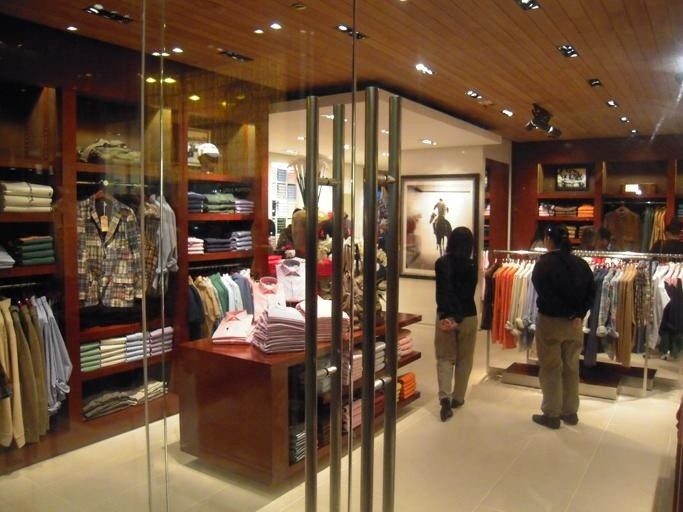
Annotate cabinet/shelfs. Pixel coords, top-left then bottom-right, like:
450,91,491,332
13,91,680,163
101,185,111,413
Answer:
533,160,683,254
177,305,423,485
1,80,272,479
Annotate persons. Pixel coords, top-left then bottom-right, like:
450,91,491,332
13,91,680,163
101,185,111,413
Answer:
278,208,303,250
434,198,449,220
435,227,478,422
532,225,595,428
527,226,618,253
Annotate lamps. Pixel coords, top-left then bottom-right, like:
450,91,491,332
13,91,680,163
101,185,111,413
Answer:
525,104,563,138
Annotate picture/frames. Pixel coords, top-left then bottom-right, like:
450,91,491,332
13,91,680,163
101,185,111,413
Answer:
397,172,480,276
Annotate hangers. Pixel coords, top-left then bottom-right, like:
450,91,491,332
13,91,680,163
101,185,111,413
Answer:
486,250,683,285
95,178,149,203
7,282,43,307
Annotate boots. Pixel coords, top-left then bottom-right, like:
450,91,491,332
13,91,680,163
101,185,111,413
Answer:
441,398,464,421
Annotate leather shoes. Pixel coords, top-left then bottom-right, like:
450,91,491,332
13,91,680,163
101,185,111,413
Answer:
533,414,578,428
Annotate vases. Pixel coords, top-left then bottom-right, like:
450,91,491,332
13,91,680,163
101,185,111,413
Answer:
290,207,329,258
